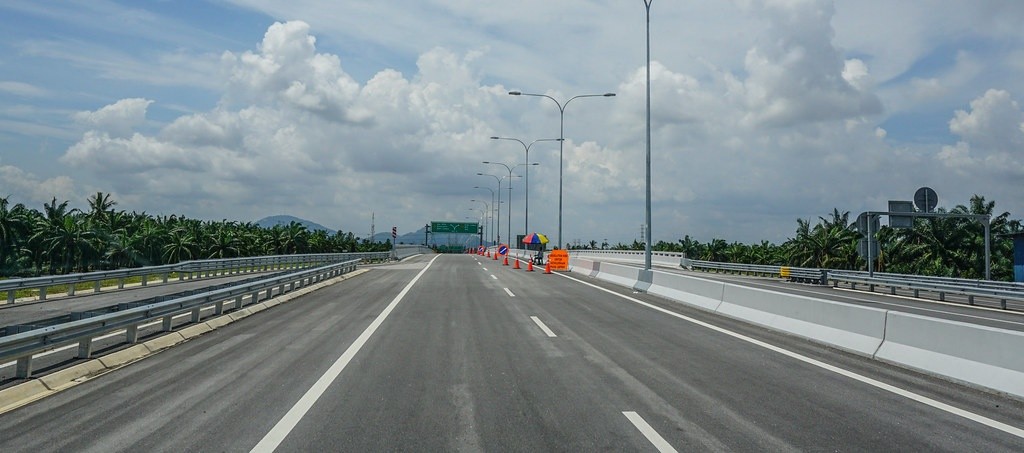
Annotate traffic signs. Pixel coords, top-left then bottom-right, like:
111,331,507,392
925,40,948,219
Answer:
431,221,479,234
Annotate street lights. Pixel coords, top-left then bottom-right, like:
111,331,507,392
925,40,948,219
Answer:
474,186,514,245
490,136,565,249
477,173,523,246
509,90,616,249
471,200,505,246
483,162,539,249
469,208,498,245
465,216,492,245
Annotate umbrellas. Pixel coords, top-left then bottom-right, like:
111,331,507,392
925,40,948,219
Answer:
522,232,549,265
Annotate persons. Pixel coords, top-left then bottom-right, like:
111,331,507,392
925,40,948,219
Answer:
532,247,543,265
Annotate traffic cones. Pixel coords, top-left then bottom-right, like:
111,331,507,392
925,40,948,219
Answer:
486,247,491,257
492,248,499,260
542,254,555,274
502,251,510,266
468,247,485,256
512,253,522,269
526,254,536,272
